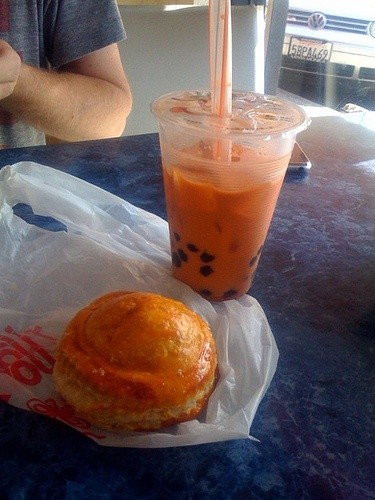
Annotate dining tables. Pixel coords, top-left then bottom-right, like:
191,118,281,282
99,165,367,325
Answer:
0,111,375,500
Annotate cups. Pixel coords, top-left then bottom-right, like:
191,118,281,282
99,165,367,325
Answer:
151,88,311,302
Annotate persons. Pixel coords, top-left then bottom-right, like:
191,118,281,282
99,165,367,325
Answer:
0,0,131,149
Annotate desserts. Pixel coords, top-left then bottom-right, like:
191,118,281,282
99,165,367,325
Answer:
54,289,219,431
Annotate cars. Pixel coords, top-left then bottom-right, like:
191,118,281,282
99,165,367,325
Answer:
272,2,375,97
